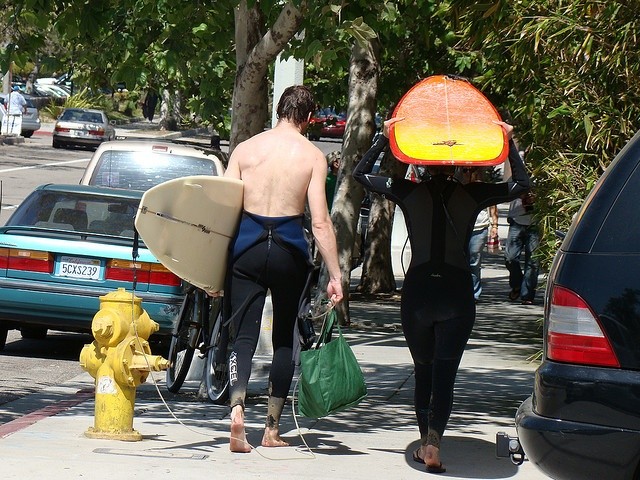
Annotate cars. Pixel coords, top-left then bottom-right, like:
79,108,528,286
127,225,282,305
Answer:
0,183,200,349
52,107,116,147
306,107,347,141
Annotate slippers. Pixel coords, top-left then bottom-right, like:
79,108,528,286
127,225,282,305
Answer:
522,297,533,304
509,284,521,301
412,447,424,461
426,461,446,473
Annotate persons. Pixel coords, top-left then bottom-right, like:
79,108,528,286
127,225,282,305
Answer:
144,89,162,124
351,117,530,474
326,151,342,213
3,85,27,136
206,86,345,453
359,134,386,260
503,196,541,305
468,203,499,304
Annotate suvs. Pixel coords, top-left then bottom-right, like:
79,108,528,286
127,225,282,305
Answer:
496,130,640,479
76,139,228,224
0,94,41,137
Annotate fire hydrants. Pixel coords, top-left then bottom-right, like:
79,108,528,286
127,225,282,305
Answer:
80,287,172,441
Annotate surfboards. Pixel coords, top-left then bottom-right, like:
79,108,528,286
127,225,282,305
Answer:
389,73,510,166
134,175,244,293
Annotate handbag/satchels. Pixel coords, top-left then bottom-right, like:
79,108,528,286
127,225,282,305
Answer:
295,304,368,419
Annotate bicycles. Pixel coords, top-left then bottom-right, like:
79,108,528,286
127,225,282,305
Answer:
167,283,230,405
